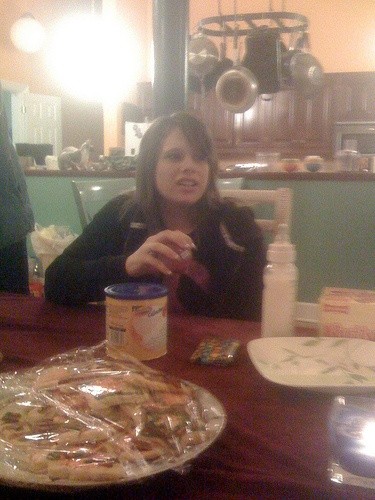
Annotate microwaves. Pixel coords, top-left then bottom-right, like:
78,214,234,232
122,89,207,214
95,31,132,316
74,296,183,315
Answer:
334,121,375,156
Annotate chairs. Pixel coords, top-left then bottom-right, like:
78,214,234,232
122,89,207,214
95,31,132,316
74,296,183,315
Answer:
73,176,292,238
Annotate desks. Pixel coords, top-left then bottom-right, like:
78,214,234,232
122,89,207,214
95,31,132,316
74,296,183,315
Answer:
0,291,375,500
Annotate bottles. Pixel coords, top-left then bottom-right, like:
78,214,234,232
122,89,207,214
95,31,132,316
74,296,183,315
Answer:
259,223,298,337
338,149,361,172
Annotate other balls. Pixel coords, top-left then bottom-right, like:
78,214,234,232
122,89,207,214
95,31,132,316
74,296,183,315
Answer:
302,155,324,172
157,240,193,273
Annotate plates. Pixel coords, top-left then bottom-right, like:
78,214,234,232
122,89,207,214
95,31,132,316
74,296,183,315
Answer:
0,378,226,488
246,336,375,395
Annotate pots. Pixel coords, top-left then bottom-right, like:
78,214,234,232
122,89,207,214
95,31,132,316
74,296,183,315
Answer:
188,23,324,115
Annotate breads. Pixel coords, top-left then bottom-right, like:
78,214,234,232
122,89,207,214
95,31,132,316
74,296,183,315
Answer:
0,357,211,481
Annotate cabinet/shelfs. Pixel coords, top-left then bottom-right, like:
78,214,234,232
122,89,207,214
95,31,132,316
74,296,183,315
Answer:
137,71,375,162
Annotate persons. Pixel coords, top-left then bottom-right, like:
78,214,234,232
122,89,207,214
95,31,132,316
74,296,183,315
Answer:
44,112,266,319
0,98,35,296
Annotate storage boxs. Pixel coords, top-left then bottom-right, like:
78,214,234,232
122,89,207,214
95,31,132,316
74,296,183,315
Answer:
318,287,375,341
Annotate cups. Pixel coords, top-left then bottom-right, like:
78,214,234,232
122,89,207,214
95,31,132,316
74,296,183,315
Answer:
327,395,375,489
255,152,322,172
